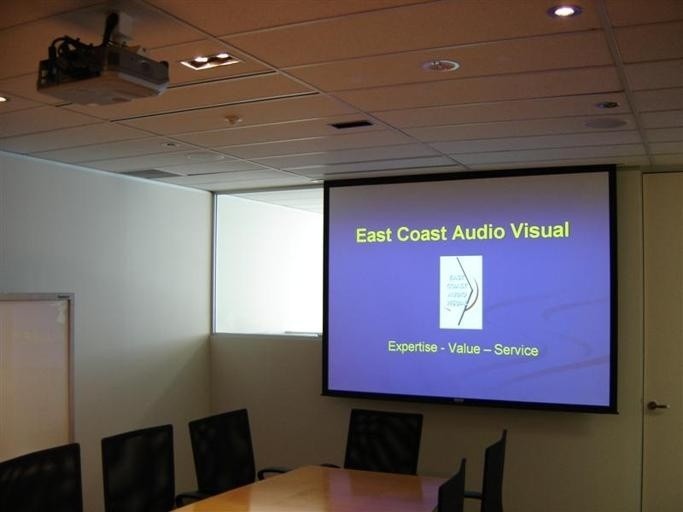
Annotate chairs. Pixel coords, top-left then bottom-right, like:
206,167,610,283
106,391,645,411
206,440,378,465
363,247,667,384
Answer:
101,424,200,512
437,458,466,512
321,408,424,476
463,427,508,512
187,408,293,495
0,442,83,512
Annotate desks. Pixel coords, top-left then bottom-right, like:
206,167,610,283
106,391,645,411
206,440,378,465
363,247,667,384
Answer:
173,465,451,512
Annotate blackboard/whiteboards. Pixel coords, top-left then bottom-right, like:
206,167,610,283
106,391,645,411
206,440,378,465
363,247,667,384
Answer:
0,292,76,465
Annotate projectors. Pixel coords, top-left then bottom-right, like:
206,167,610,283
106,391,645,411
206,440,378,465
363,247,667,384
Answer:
35,12,170,106
